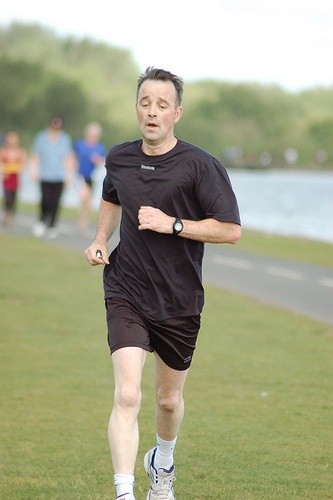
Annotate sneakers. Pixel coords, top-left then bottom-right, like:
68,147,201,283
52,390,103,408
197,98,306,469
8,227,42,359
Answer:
143,447,176,500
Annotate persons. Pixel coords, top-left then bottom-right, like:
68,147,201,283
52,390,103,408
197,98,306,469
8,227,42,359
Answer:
30,115,76,240
0,130,27,225
86,67,241,500
73,123,106,235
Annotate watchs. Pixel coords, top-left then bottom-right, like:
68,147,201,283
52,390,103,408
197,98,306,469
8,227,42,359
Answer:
172,217,184,235
